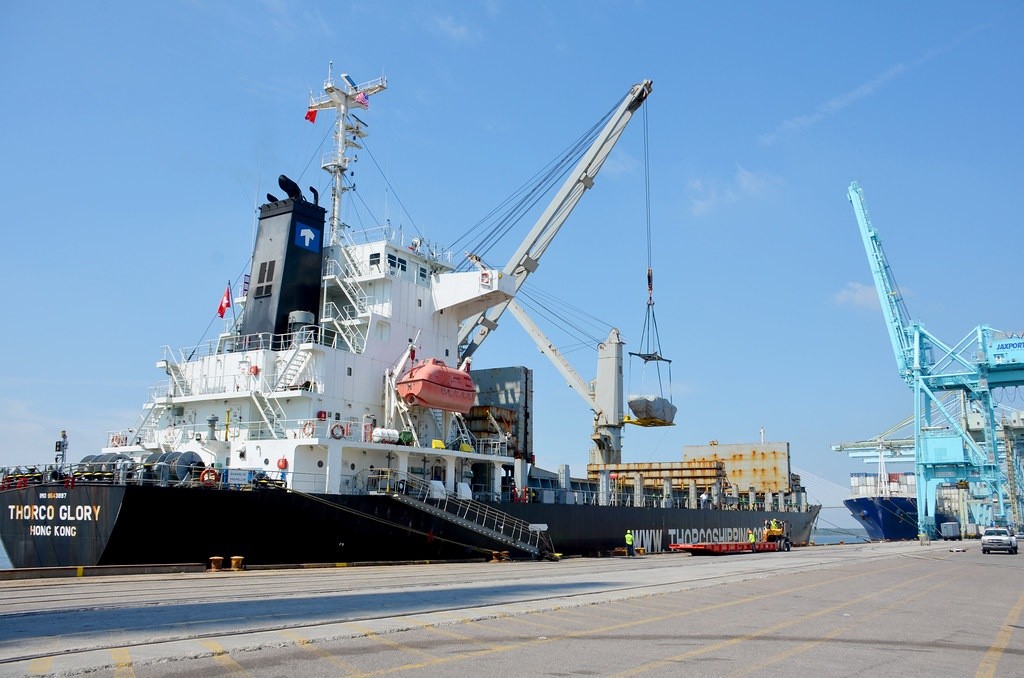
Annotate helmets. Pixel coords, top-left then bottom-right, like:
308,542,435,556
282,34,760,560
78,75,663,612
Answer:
627,530,632,533
748,530,752,533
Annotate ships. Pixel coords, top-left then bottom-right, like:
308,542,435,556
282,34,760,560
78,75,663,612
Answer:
0,60,823,569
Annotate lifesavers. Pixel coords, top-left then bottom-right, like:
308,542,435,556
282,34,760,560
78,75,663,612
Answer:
200,467,219,487
301,421,316,437
330,424,345,440
110,434,121,447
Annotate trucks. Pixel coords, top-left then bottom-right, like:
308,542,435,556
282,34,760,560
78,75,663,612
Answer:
668,521,792,555
967,524,986,539
941,522,962,541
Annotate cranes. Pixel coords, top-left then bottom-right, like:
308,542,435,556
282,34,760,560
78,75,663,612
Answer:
830,180,1024,538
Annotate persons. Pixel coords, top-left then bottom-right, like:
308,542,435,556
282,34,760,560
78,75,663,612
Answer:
700,491,708,509
625,530,635,557
747,530,757,553
772,518,777,529
714,493,718,510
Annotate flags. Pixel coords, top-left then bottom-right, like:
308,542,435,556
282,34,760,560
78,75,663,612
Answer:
305,110,318,123
218,288,231,318
357,92,369,106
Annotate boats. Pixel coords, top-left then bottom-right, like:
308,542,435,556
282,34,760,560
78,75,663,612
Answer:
396,357,477,413
842,496,982,541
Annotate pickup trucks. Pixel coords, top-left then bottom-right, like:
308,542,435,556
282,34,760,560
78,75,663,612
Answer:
981,525,1018,554
